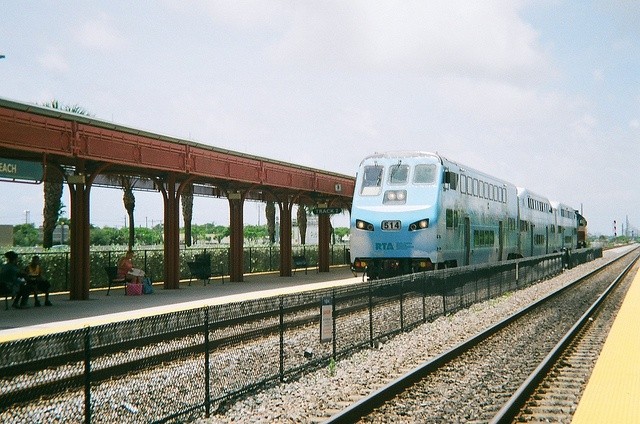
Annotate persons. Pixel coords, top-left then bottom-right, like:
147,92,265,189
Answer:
24,255,53,307
0,251,29,309
117,250,143,284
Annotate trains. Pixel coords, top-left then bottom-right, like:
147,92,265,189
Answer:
350,151,587,281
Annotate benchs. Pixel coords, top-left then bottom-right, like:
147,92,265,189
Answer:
189,262,225,287
105,265,153,296
1,274,49,309
292,256,319,276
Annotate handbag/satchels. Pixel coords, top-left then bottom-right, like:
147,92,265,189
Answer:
143,278,153,294
